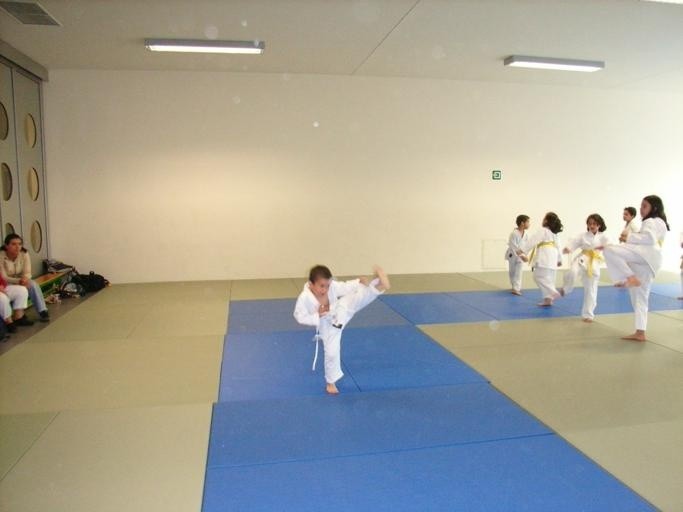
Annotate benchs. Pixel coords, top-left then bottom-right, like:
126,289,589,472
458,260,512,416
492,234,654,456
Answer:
29,267,79,297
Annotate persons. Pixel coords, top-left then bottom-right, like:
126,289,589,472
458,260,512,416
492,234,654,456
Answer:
677,241,683,299
603,196,670,341
293,265,391,394
619,207,639,244
0,274,34,333
560,214,606,323
517,212,564,306
0,234,49,321
505,215,529,295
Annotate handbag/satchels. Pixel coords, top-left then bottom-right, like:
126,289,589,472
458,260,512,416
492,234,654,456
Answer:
72,272,108,293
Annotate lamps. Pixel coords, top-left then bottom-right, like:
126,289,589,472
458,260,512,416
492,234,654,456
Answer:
142,37,265,58
503,56,605,74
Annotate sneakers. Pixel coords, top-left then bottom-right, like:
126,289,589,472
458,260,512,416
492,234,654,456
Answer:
6,311,51,333
